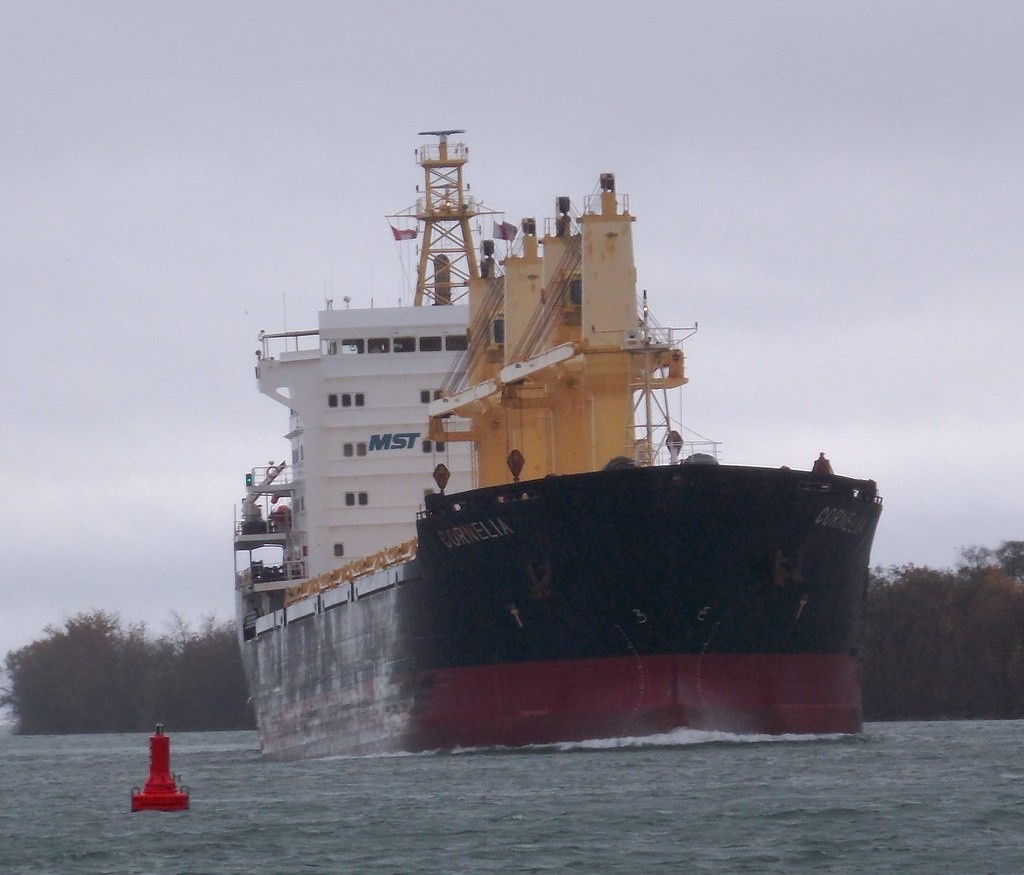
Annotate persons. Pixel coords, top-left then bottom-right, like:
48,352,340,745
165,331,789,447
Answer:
812,453,834,475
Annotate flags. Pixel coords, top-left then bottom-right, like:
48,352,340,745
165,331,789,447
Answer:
493,222,517,241
391,225,416,240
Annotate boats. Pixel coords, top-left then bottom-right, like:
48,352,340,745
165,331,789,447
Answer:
229,126,887,765
